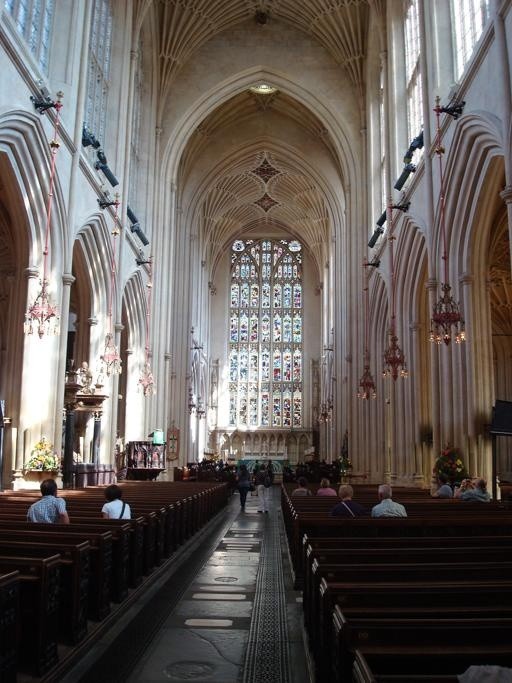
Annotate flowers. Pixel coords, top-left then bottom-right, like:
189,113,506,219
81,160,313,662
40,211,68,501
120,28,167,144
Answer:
18,435,62,473
432,448,467,482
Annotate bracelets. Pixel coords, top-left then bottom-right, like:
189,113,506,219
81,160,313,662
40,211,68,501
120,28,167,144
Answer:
459,484,464,487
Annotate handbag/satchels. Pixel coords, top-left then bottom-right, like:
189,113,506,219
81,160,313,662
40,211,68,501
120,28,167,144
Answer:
248,484,256,492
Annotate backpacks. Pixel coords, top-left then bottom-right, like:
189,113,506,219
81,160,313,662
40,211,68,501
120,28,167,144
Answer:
263,475,272,488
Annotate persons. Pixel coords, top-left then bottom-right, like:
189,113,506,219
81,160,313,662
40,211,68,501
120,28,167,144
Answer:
454,478,490,502
428,474,453,498
370,484,408,517
317,478,338,496
332,485,364,518
102,484,132,520
26,479,71,525
292,478,311,495
190,456,276,515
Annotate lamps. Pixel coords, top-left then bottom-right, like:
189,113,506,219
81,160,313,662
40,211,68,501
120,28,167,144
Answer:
90,190,127,376
80,127,150,247
381,197,415,382
131,249,157,397
430,90,476,344
356,259,382,402
187,345,206,418
316,346,335,424
367,130,424,250
23,86,68,341
